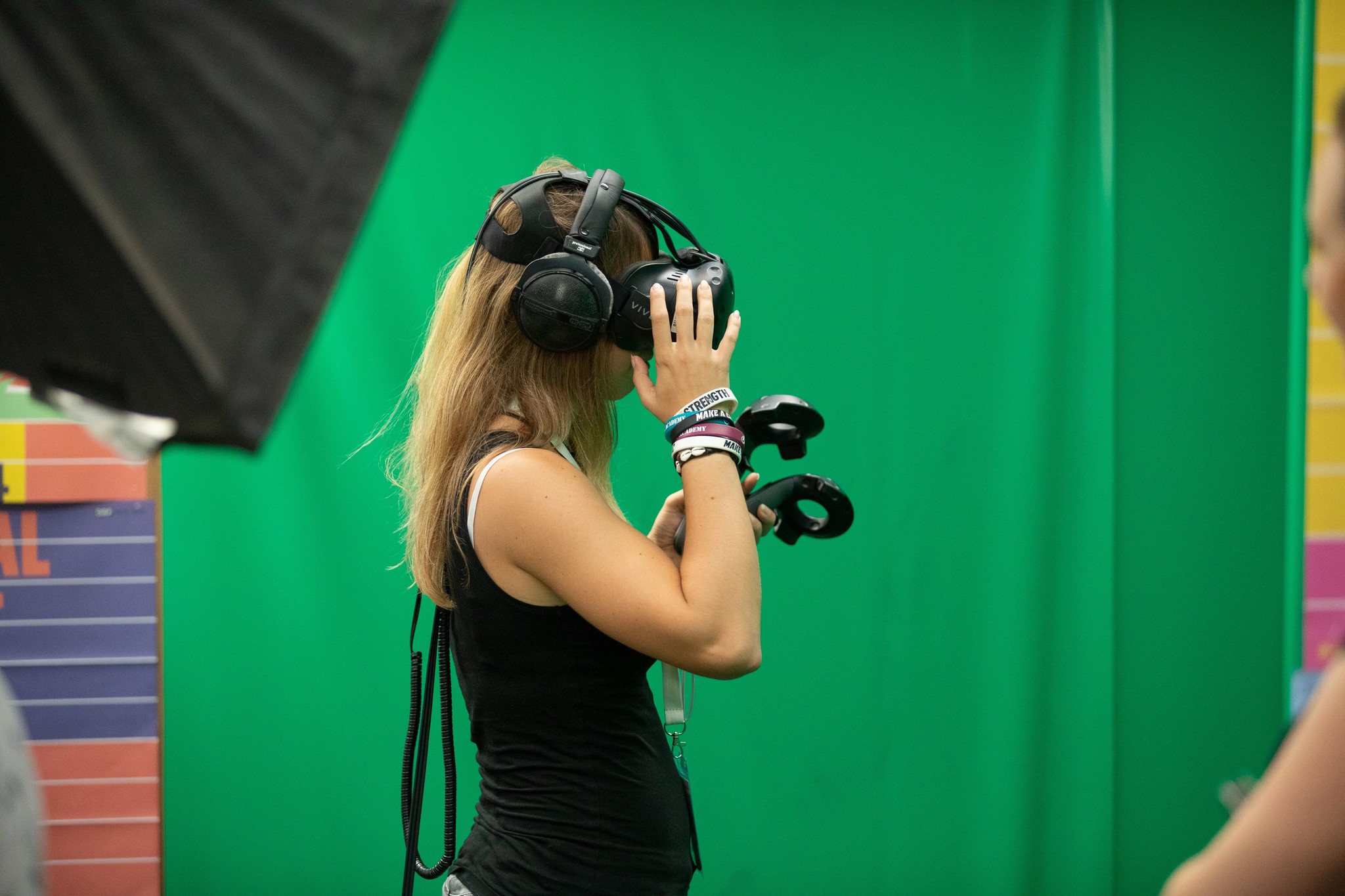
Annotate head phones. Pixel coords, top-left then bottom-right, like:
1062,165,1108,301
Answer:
510,167,625,353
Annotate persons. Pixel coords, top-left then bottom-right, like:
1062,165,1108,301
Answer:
1149,95,1345,895
404,163,776,896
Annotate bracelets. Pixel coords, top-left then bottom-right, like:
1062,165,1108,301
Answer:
663,388,745,474
